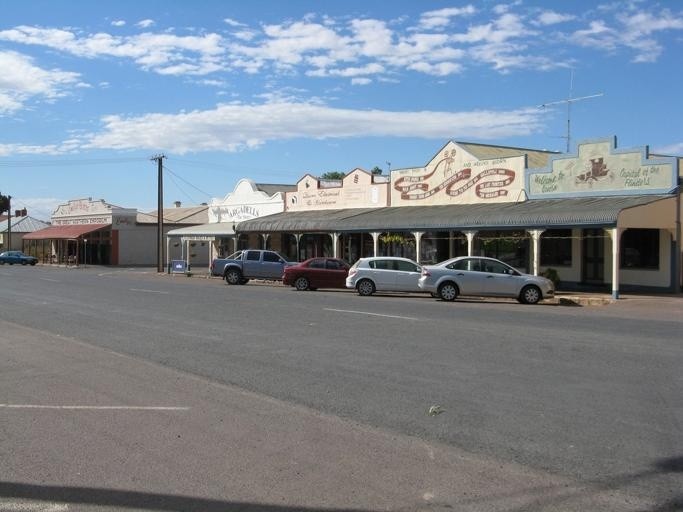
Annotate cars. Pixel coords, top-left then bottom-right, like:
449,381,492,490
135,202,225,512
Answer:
0,251,38,265
